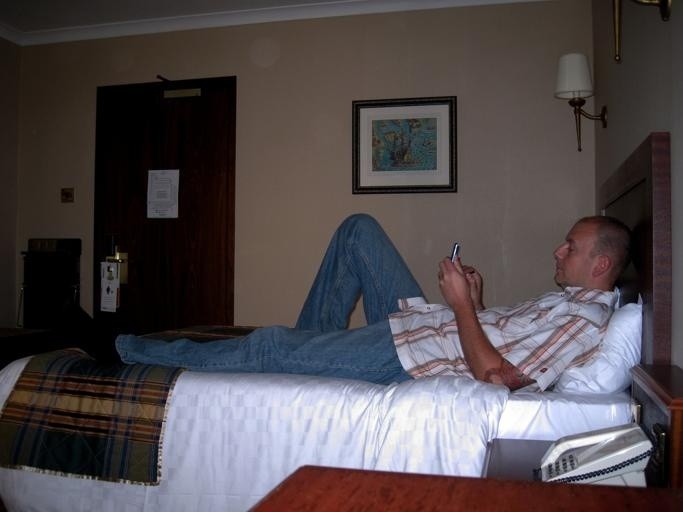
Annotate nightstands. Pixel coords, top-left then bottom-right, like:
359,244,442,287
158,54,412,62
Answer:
486,438,658,494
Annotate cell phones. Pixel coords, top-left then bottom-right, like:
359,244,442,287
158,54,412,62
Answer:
451,243,460,262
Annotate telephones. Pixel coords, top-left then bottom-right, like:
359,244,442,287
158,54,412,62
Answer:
540,422,654,487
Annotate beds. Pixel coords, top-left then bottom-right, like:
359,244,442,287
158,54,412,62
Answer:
0,133,670,512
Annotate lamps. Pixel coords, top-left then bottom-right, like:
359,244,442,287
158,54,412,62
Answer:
555,52,607,153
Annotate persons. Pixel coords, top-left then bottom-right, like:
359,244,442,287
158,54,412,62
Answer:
54,211,634,394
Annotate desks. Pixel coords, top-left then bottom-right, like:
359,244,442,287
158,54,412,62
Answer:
251,467,676,511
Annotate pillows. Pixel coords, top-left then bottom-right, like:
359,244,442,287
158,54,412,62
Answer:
552,293,644,397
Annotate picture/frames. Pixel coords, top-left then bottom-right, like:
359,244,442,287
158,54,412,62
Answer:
350,96,459,194
